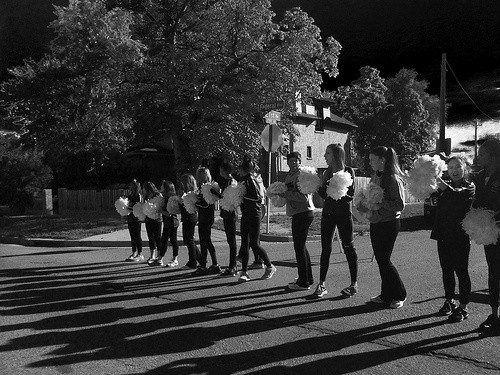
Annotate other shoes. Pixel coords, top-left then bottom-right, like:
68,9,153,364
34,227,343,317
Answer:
448,306,469,322
479,313,500,335
248,262,264,268
261,264,276,280
438,299,457,315
126,253,145,261
288,282,310,290
370,295,389,303
312,283,327,298
341,285,359,298
152,259,163,265
192,265,221,276
166,259,178,267
389,297,406,308
181,264,197,269
237,271,250,282
216,268,239,277
293,278,315,284
147,256,158,263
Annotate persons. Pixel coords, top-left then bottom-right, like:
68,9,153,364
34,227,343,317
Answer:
429,156,475,323
116,155,276,283
274,152,313,289
357,146,408,308
314,144,359,298
471,138,500,334
